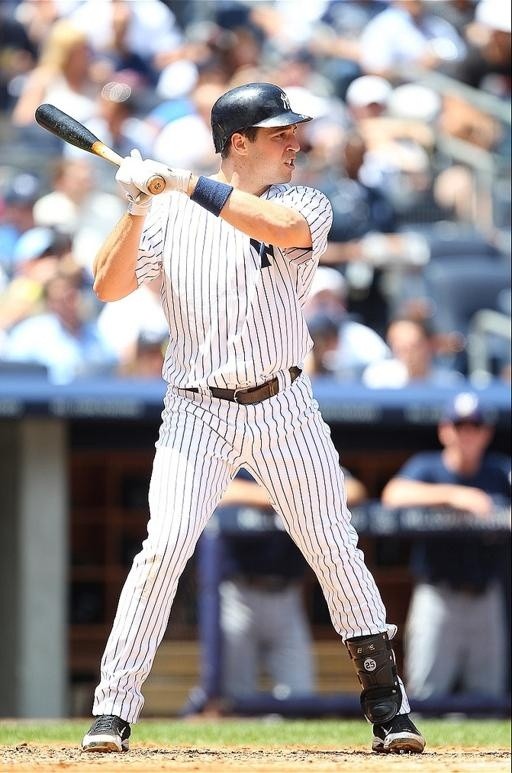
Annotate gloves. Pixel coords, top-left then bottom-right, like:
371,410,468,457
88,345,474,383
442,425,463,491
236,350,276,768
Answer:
132,158,193,196
114,148,154,216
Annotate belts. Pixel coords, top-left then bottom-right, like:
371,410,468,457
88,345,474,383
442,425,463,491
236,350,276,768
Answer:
178,366,303,405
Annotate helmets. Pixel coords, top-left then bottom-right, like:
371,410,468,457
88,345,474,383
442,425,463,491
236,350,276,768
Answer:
209,82,315,153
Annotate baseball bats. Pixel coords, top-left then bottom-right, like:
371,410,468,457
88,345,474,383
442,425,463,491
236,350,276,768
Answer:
35,104,166,194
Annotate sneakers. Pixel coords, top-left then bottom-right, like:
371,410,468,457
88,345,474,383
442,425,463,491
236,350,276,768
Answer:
81,714,131,754
371,713,426,754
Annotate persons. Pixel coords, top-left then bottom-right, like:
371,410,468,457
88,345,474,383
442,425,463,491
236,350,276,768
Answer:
3,0,510,388
207,461,366,701
377,387,511,718
84,79,429,753
